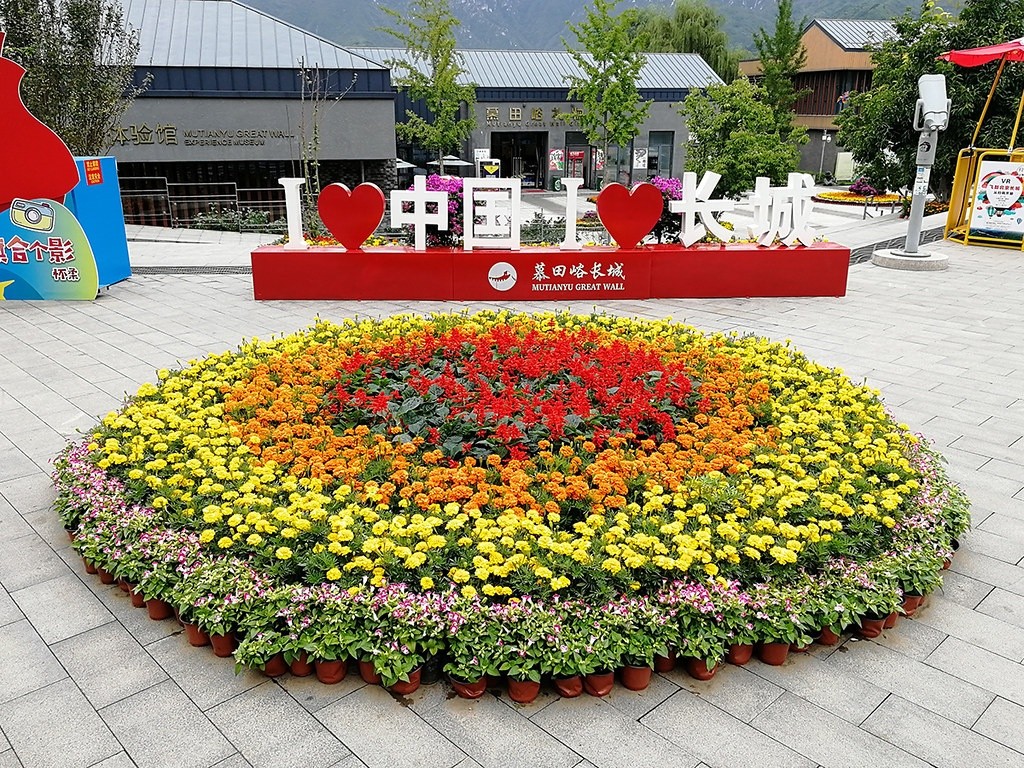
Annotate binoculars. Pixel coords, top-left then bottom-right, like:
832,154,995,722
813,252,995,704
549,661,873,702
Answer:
918,74,948,132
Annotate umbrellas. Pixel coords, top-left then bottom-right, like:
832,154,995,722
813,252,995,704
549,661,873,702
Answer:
427,155,474,165
396,157,417,168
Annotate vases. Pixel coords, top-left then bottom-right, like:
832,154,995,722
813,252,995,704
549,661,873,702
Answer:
72,532,949,702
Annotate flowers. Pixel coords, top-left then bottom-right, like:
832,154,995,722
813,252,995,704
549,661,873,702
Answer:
172,174,799,246
50,310,975,685
809,179,950,220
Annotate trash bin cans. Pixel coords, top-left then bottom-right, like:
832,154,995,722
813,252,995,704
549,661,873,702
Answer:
552,176,560,192
597,176,603,191
